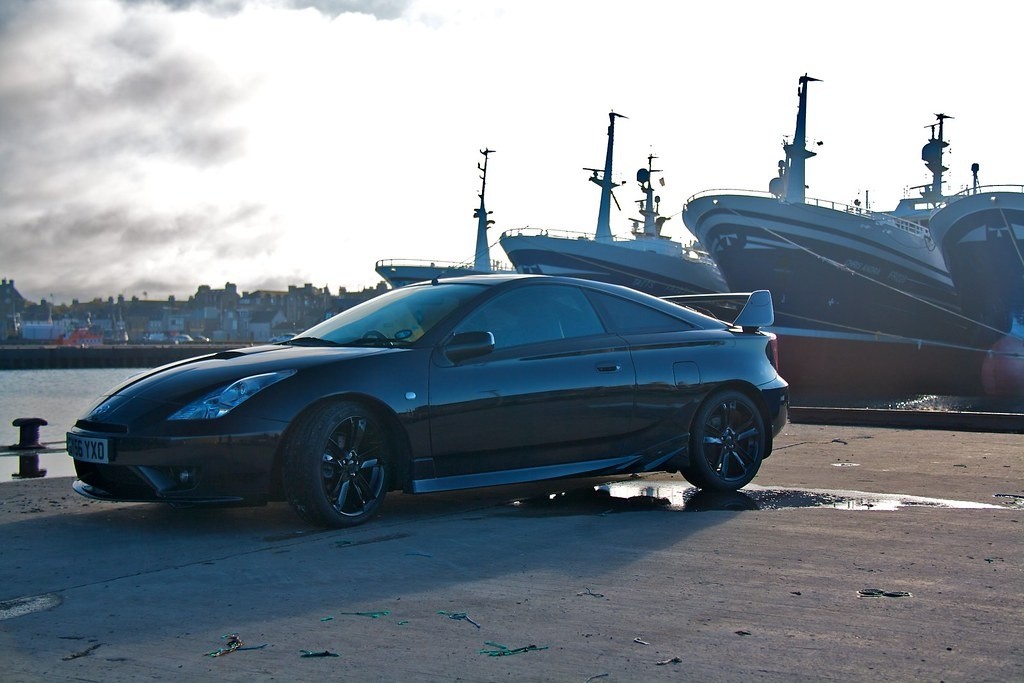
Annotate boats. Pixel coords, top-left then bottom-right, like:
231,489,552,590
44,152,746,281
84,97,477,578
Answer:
374,73,1023,409
46,322,106,344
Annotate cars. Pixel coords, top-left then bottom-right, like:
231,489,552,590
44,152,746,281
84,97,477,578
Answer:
268,334,296,343
192,335,211,342
170,335,195,343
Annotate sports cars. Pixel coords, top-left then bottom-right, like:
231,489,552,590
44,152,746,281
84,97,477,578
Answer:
65,271,789,528
